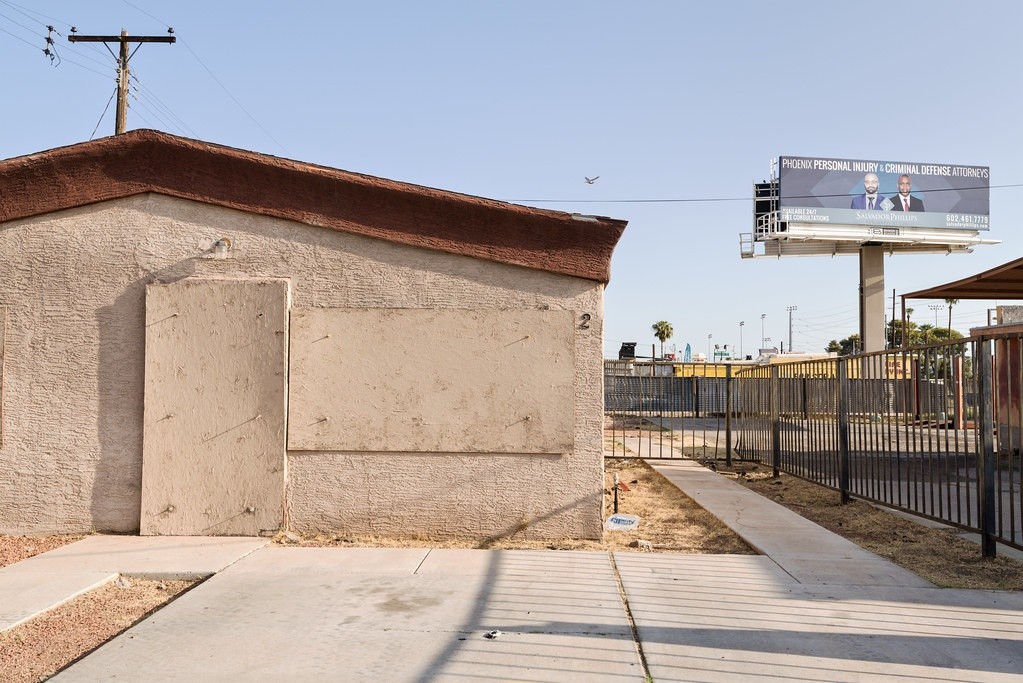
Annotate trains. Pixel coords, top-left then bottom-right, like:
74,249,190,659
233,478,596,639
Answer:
603,375,949,418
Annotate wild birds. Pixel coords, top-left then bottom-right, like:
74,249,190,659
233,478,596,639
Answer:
584,176,599,184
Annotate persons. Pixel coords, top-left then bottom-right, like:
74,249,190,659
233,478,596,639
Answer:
850,172,890,212
887,173,925,212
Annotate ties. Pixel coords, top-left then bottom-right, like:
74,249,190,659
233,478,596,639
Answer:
904,199,910,211
868,197,875,210
